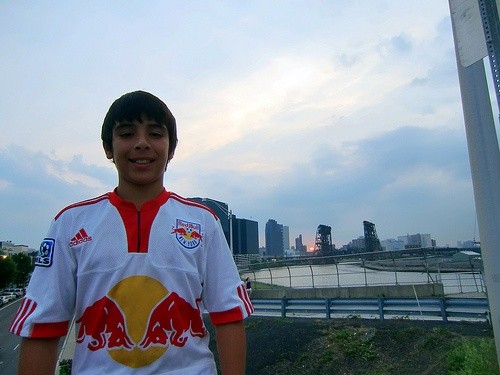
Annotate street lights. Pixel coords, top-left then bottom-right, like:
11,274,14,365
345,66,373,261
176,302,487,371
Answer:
201,197,234,257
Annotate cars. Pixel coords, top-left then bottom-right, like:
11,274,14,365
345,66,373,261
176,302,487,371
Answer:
2,292,16,300
0,295,8,303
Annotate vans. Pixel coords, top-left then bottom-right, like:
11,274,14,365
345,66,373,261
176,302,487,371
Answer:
4,288,23,297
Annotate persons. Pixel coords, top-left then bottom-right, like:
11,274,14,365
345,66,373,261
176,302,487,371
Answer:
7,91,255,375
245,277,253,301
